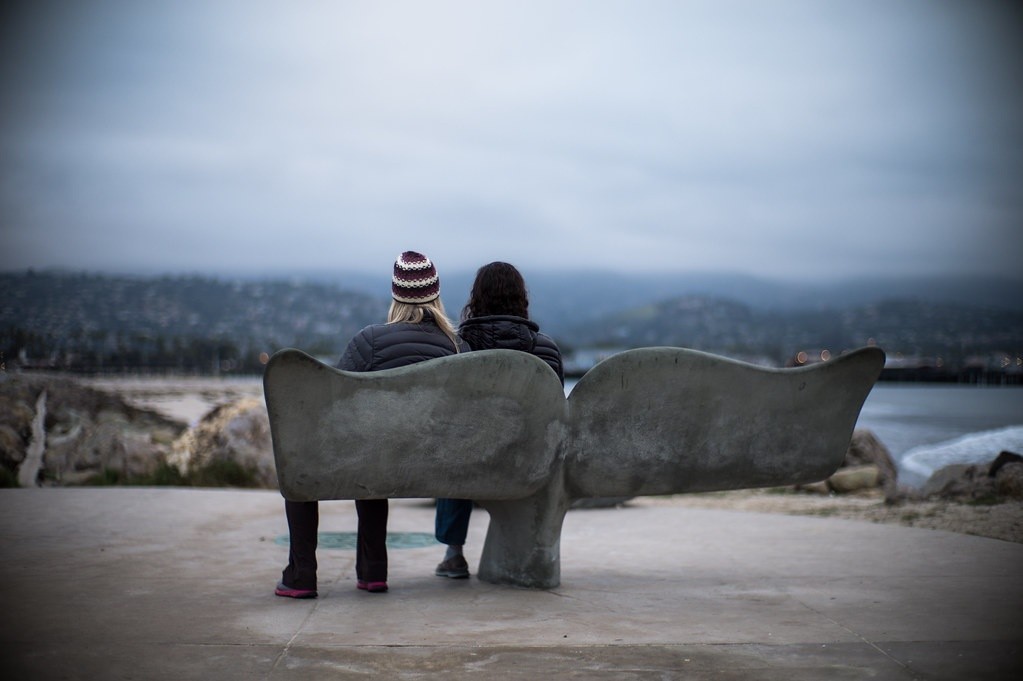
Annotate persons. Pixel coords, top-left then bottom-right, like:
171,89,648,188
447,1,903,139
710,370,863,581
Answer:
434,261,563,577
274,250,471,600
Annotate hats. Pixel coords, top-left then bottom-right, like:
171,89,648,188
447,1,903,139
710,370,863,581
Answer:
391,252,440,303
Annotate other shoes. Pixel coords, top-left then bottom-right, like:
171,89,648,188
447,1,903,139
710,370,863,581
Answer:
435,555,468,579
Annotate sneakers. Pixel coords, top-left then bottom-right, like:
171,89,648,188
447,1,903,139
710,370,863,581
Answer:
274,580,318,600
356,571,388,593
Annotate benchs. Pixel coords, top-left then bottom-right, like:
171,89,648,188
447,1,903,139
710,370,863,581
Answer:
262,348,885,591
